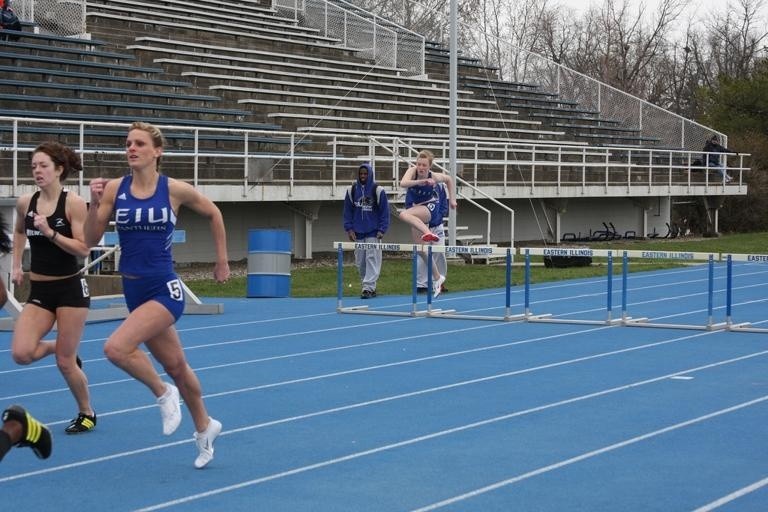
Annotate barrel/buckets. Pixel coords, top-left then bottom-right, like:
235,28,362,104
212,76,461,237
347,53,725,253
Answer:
246,226,293,297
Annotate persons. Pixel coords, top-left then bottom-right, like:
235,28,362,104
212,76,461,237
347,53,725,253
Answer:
398,149,458,299
342,163,390,299
83,121,231,468
0,0,14,41
0,208,52,462
703,135,738,183
11,140,96,432
405,182,450,293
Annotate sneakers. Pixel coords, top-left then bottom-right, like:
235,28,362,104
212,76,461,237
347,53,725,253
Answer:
432,274,446,298
193,416,222,468
156,381,182,436
1,403,53,459
65,408,97,434
360,291,377,299
421,233,439,242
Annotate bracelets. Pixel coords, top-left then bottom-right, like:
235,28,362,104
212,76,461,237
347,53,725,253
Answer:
52,230,59,242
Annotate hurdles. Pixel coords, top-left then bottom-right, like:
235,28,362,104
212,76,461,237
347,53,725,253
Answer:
85,234,225,321
333,240,768,335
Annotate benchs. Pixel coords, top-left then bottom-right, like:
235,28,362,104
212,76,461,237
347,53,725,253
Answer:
0,0,711,185
